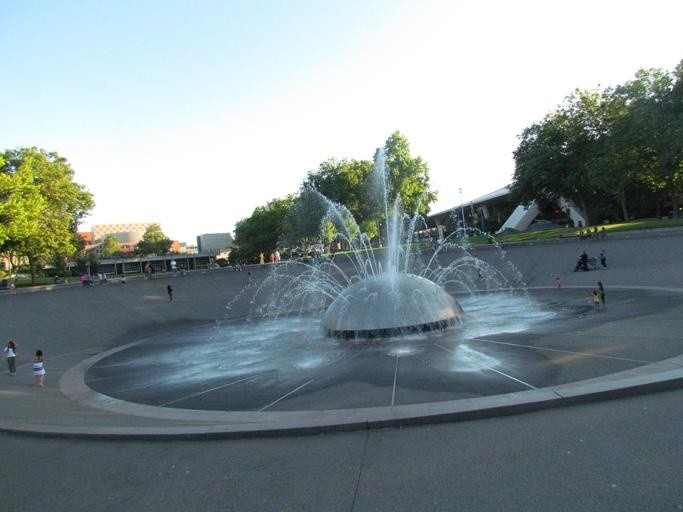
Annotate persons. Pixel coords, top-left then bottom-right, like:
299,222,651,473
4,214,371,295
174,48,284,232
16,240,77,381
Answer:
555,277,561,289
268,242,324,264
32,350,47,386
598,281,605,304
592,290,600,311
79,271,127,287
144,260,185,279
579,227,607,241
573,250,607,272
3,340,20,376
168,284,174,303
